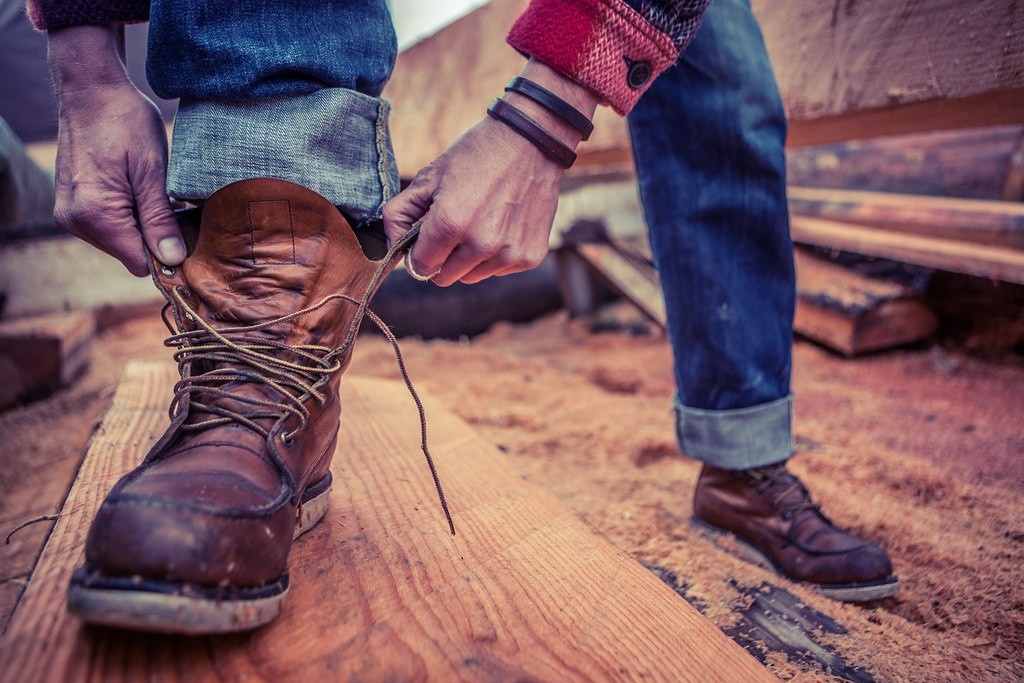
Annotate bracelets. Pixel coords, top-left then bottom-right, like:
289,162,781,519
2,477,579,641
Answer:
487,76,593,168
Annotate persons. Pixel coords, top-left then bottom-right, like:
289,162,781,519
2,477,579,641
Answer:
30,0,898,635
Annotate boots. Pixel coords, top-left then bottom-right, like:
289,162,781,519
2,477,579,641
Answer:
64,175,455,637
689,458,901,607
